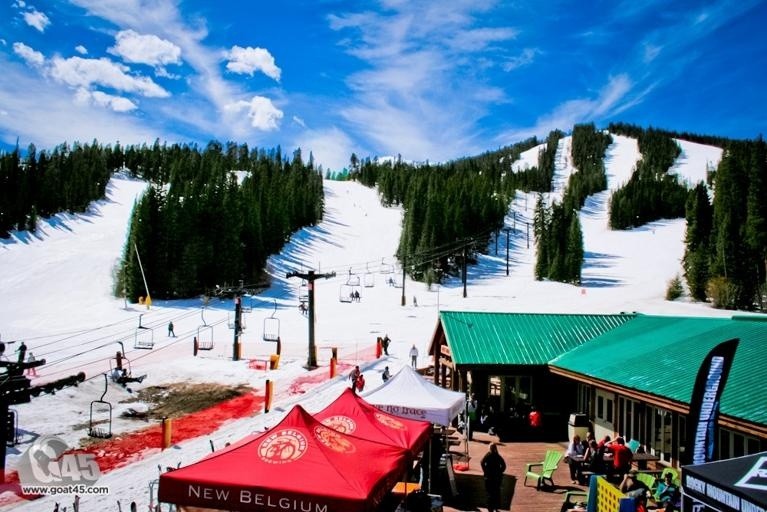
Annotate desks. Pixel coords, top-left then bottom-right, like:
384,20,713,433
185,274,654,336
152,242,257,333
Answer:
568,454,659,470
390,424,461,496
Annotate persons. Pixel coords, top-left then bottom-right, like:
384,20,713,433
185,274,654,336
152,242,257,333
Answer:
112,368,127,380
409,344,418,367
480,443,506,512
565,432,633,484
15,342,36,375
467,392,478,441
422,427,442,494
168,322,176,337
349,366,365,392
376,336,390,358
382,366,390,382
620,474,680,512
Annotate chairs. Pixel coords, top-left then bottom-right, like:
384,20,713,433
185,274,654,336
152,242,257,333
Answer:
524,448,564,491
565,468,681,512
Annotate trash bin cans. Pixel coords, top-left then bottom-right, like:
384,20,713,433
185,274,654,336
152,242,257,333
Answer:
568,413,590,443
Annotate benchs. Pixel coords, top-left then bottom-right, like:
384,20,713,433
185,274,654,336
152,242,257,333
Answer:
581,470,663,479
